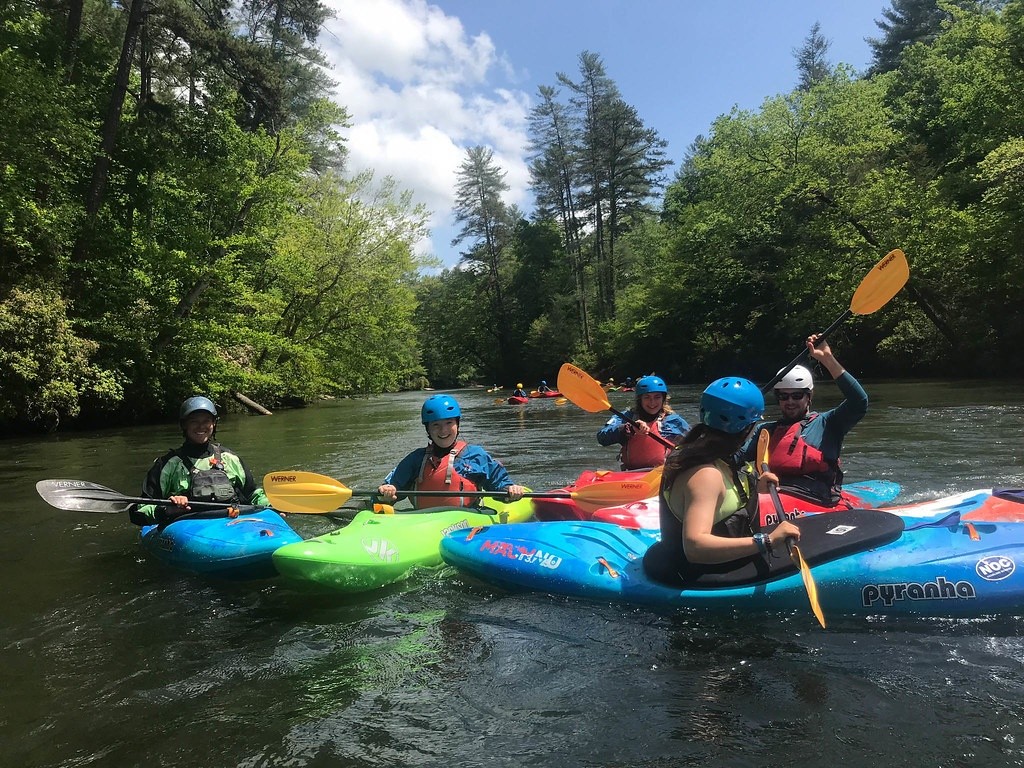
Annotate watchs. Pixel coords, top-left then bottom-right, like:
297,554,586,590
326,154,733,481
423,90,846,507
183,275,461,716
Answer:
753,533,766,553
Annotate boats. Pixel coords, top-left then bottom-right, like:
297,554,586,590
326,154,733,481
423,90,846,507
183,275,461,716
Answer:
589,471,901,543
506,396,528,406
493,388,500,392
139,498,307,577
440,489,1023,621
530,390,573,404
270,486,537,592
531,464,676,526
623,387,635,393
607,386,619,393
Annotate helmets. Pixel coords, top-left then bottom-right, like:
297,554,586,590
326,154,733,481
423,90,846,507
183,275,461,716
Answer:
634,376,667,399
517,383,523,389
179,396,217,425
699,376,765,433
773,365,813,394
541,381,546,387
642,375,647,378
421,394,462,423
609,378,615,382
627,377,631,382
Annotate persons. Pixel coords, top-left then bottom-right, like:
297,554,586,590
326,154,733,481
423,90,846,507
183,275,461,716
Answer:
493,384,497,389
129,396,287,526
659,377,801,565
734,334,869,486
597,376,692,472
624,377,632,388
605,377,614,388
377,394,523,510
512,382,527,397
537,381,552,393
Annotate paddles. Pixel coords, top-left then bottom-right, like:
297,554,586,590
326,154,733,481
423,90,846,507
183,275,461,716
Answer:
642,247,913,498
650,372,655,376
555,361,675,450
636,377,641,382
754,426,829,631
34,476,375,528
595,380,606,386
557,380,601,405
495,395,513,402
530,391,538,396
259,470,653,518
487,386,503,393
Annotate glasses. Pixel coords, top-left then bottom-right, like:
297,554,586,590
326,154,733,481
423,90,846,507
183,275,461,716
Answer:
778,392,807,402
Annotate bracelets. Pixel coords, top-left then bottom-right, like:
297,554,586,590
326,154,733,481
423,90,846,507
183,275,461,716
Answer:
764,534,780,567
